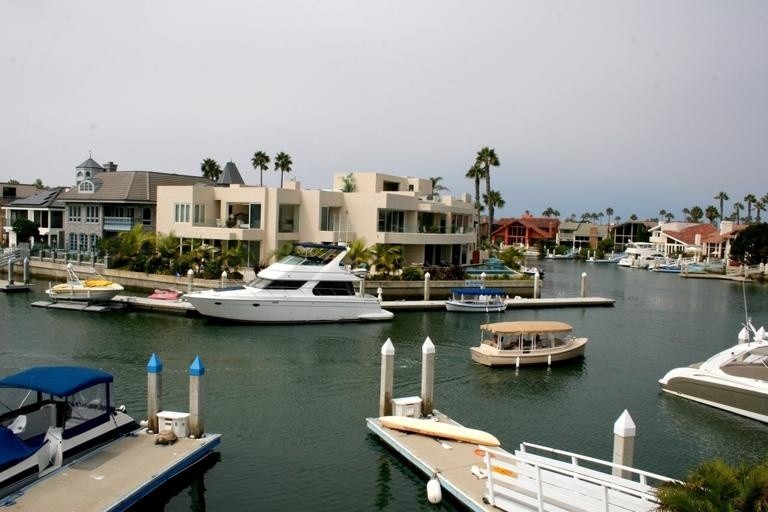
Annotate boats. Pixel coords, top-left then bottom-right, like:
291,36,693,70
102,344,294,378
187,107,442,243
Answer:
183,242,394,325
0,366,142,501
444,288,508,312
44,266,125,304
470,320,588,368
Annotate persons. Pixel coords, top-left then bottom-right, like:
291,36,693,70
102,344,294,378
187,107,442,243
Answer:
516,335,526,346
501,336,505,349
491,334,498,346
536,334,541,345
